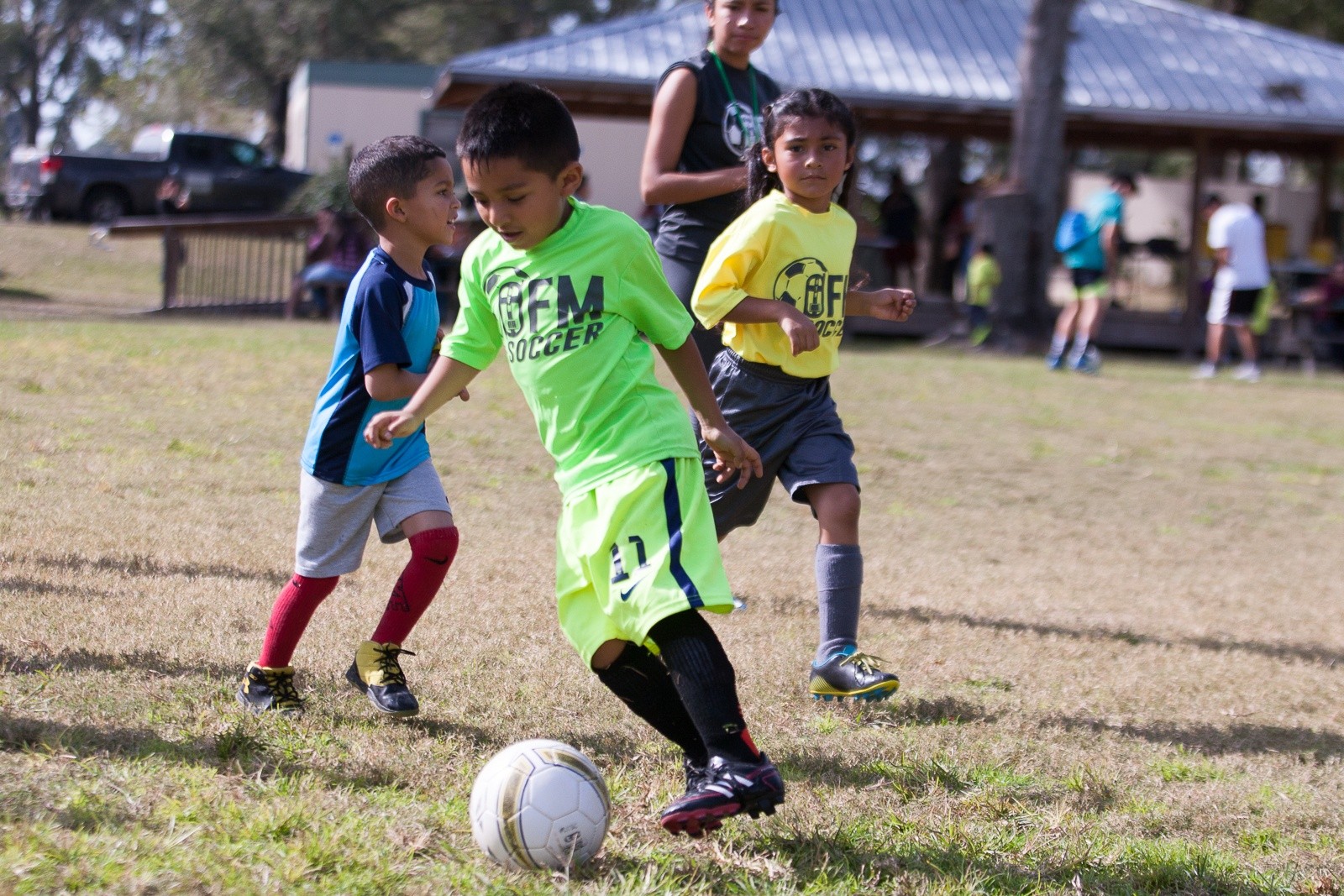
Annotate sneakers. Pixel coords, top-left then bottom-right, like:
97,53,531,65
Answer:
660,752,784,839
236,661,309,724
809,645,900,702
345,641,419,718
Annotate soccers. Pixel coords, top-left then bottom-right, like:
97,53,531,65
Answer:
469,737,612,873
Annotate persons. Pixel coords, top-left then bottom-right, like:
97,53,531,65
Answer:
364,81,785,837
285,205,477,319
642,1,784,372
685,87,918,702
238,133,470,716
842,158,1272,382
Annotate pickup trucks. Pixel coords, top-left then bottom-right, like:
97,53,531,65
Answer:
8,132,314,222
